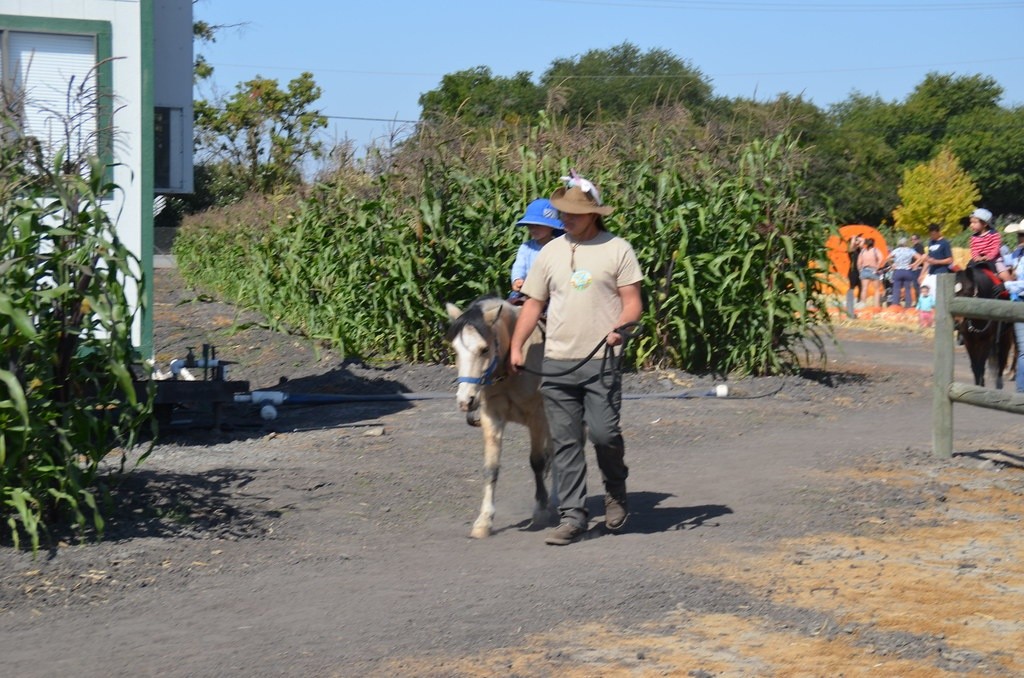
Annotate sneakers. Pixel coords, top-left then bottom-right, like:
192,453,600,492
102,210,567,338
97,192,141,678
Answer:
544,522,589,544
604,481,630,529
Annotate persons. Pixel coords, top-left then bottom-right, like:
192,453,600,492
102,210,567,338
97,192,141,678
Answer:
848,209,1024,391
509,198,564,318
505,184,643,546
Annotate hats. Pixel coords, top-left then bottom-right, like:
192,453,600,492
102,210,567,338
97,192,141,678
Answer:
969,208,994,229
549,169,616,215
516,199,564,230
1004,218,1024,233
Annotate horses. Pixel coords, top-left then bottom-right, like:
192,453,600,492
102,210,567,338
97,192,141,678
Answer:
445,298,556,537
947,263,1017,390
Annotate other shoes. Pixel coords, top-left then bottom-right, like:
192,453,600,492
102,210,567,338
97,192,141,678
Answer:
1009,293,1021,302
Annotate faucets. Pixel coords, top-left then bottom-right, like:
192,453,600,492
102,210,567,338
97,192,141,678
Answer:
168,342,240,381
